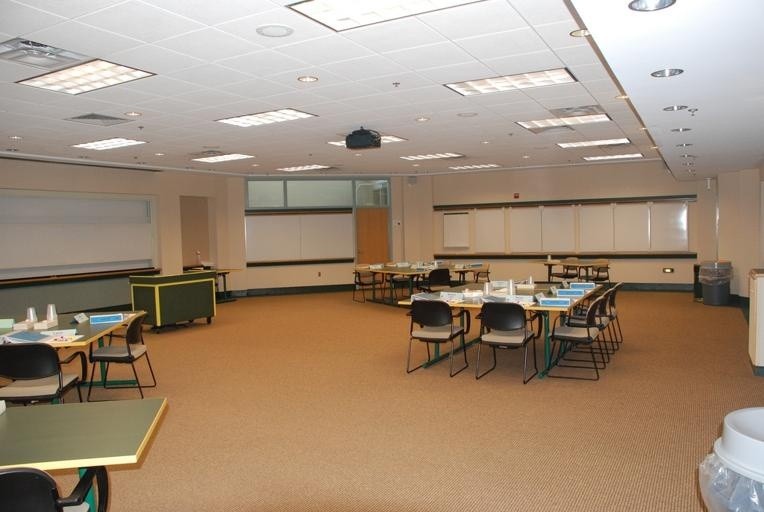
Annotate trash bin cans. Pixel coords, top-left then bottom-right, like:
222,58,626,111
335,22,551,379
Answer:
713,407,764,512
700,261,733,306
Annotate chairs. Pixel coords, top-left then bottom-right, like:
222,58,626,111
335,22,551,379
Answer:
82,307,159,403
0,335,92,409
0,465,111,512
351,256,629,386
0,318,16,336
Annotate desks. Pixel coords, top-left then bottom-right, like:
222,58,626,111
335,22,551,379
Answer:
1,309,147,404
0,397,176,512
127,270,218,335
194,266,244,306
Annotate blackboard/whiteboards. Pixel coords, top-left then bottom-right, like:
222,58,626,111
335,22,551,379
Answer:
433,194,698,259
244,206,355,266
0,189,161,284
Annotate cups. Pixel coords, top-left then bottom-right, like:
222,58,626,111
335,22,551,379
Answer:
25,305,36,321
507,279,515,295
433,261,438,269
483,282,492,297
527,276,533,285
46,302,57,320
547,255,551,264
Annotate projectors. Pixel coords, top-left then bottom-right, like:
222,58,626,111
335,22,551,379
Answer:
346,129,381,149
